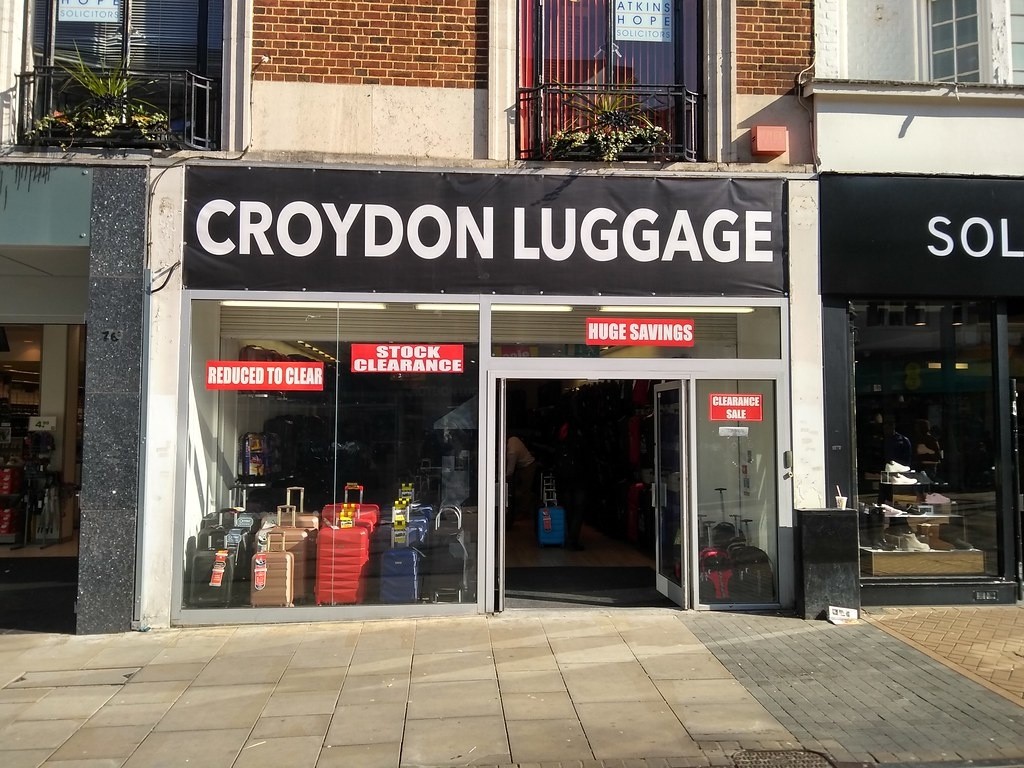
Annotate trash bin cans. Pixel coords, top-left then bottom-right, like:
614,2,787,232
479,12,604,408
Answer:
793,508,861,620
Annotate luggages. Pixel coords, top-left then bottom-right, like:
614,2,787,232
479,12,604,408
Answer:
622,379,681,557
236,344,335,513
677,488,776,603
532,471,566,549
190,457,441,605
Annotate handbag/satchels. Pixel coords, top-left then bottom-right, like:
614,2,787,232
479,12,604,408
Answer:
428,506,467,590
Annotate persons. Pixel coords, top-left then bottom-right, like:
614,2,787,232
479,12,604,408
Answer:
505,435,537,522
875,416,912,513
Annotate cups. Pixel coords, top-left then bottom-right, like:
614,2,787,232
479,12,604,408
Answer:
835,496,847,510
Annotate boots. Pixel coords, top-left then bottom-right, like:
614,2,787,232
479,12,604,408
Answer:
858,513,973,551
